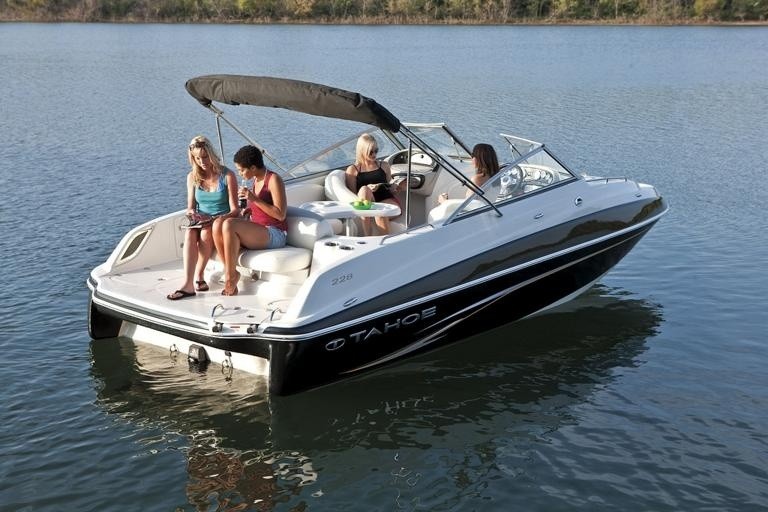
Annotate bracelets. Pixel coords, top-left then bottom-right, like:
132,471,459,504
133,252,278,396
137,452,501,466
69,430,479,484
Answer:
227,212,233,217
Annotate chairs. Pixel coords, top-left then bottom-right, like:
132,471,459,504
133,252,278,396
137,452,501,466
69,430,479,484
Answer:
323,168,411,233
282,183,357,238
428,198,488,224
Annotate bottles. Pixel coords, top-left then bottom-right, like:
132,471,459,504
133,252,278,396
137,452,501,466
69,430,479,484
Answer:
238,179,248,209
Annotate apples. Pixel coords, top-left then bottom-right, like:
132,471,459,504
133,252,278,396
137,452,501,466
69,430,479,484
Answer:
354,198,370,206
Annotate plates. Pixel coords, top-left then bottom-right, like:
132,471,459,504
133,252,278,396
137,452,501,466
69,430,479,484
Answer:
349,202,374,210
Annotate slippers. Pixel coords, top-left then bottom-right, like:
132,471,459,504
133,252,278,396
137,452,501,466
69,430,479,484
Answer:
196,280,209,291
167,290,195,300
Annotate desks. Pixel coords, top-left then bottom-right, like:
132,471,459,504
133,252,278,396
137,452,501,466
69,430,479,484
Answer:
298,199,402,235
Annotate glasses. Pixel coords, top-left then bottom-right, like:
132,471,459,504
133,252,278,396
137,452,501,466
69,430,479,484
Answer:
190,141,207,149
369,148,378,155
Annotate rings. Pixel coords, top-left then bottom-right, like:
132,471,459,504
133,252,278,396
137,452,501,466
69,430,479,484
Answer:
241,191,244,193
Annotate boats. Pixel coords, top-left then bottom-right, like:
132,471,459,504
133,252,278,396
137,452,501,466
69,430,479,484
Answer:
88,74,670,395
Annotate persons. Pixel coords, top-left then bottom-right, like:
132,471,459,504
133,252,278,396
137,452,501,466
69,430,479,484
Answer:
210,144,290,297
166,135,239,300
344,132,403,236
437,142,502,212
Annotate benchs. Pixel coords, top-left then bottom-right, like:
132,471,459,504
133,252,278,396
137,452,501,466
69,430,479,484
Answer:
189,206,332,294
